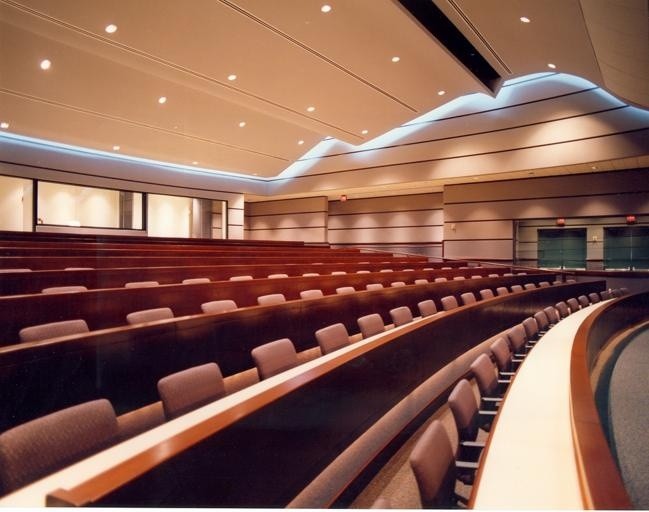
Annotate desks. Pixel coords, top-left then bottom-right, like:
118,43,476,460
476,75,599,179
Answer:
463,292,649,508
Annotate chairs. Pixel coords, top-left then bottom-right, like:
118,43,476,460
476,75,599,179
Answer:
0,258,628,508
